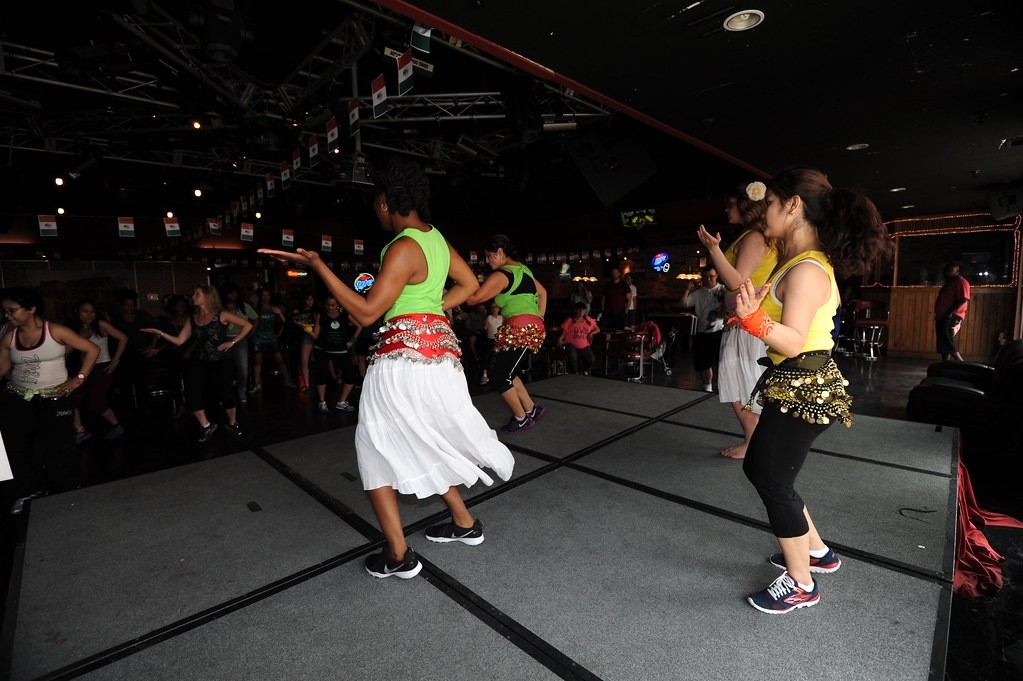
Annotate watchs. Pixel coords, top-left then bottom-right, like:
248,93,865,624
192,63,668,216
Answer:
77,373,87,381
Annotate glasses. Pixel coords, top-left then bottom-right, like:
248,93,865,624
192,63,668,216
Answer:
0,304,23,314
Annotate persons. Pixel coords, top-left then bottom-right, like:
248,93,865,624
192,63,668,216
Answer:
730,166,897,615
933,260,971,362
697,181,778,460
257,158,515,579
0,236,882,523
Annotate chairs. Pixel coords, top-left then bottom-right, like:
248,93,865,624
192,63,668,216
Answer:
837,297,890,362
525,322,679,384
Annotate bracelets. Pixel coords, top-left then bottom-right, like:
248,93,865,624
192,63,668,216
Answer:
590,331,593,336
231,340,236,344
160,332,164,337
111,357,119,365
350,336,357,343
687,288,690,291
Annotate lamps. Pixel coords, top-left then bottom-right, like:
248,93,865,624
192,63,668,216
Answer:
904,27,942,73
845,124,869,151
722,1,765,31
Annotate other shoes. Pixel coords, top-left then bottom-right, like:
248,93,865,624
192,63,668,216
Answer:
284,384,298,389
248,384,262,395
174,404,186,420
224,423,248,441
555,370,591,376
316,401,330,415
72,431,92,443
104,425,123,438
197,422,218,444
479,377,489,385
336,401,355,411
700,380,715,398
298,386,310,394
11,486,44,513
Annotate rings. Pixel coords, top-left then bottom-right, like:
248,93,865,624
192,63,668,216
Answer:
744,301,750,305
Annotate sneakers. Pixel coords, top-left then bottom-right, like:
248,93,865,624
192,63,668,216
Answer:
770,548,841,573
502,404,545,433
747,570,820,615
365,544,422,579
426,519,485,545
